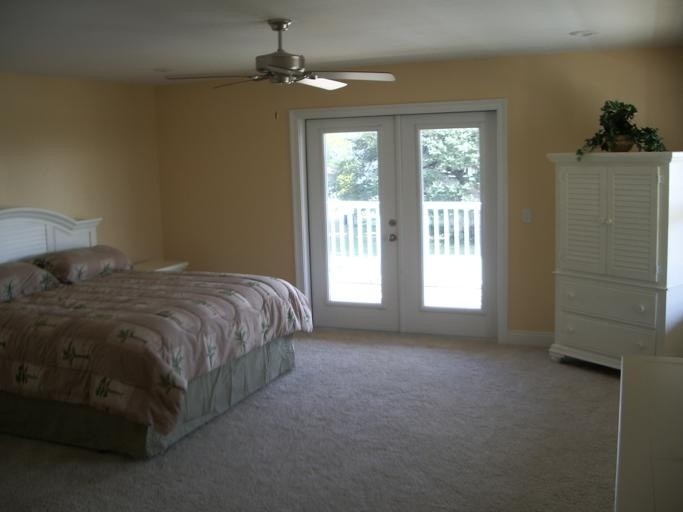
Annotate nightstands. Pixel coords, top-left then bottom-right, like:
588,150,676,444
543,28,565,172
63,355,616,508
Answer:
131,256,189,272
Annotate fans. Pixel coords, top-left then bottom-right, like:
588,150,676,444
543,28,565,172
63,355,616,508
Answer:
166,18,396,93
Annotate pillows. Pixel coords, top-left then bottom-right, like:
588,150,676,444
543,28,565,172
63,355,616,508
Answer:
0,244,128,304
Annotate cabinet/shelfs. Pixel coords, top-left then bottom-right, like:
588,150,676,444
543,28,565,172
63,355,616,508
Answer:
545,151,683,369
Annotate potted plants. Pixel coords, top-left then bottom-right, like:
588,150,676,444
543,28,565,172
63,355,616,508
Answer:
574,99,668,162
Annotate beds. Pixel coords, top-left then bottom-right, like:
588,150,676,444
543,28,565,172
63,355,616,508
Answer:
0,206,296,460
